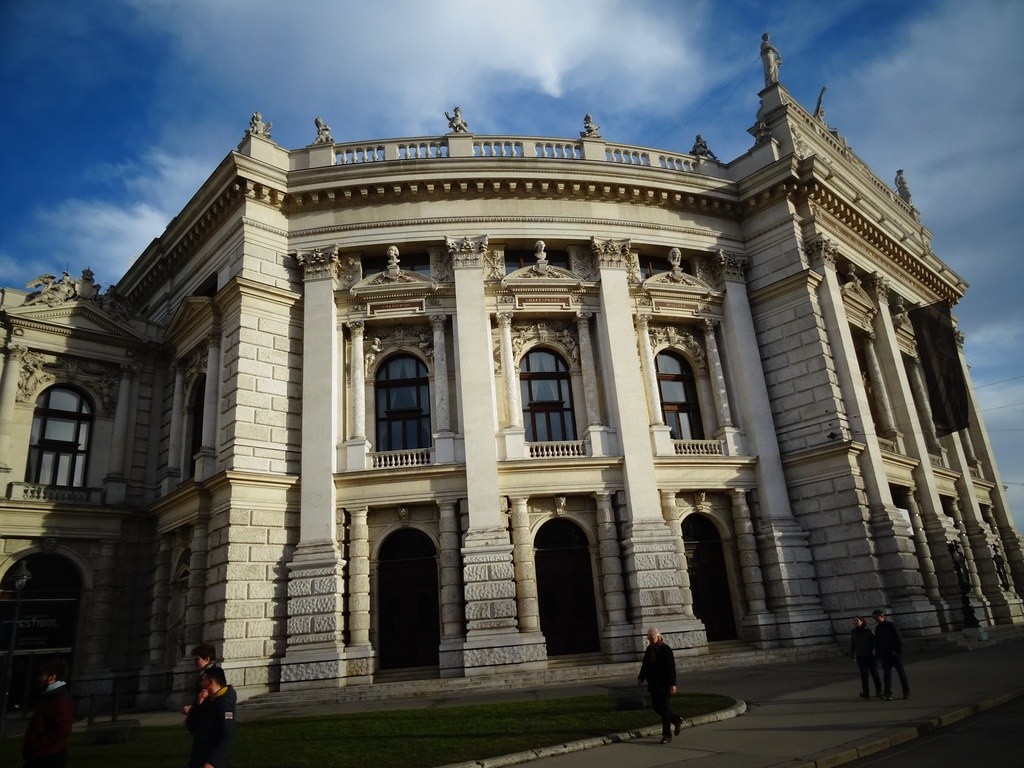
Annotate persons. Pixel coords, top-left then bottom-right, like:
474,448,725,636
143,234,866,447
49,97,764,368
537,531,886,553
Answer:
850,610,881,700
847,264,857,281
314,116,332,142
872,609,910,701
895,169,911,203
253,112,270,136
23,276,74,306
513,330,525,364
364,337,382,376
101,286,137,328
637,627,682,745
534,241,546,259
894,296,905,312
182,647,237,768
687,335,703,363
387,246,400,263
760,33,780,86
694,135,717,159
668,248,681,267
584,115,598,136
444,107,467,133
556,330,579,364
21,661,73,768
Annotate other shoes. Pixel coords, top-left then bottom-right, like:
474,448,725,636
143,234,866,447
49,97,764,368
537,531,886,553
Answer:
860,692,869,698
674,717,683,736
887,695,893,701
904,691,910,699
660,735,672,744
875,689,882,697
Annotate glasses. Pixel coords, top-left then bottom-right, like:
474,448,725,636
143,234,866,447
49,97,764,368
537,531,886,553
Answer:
647,636,657,639
